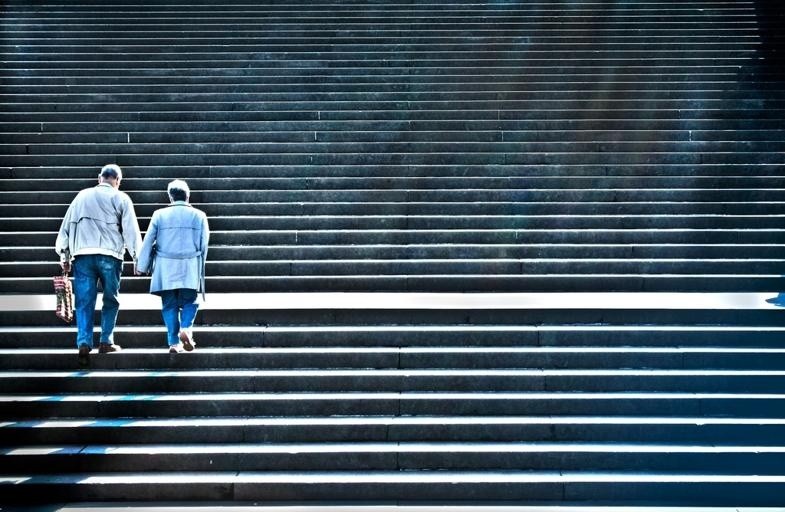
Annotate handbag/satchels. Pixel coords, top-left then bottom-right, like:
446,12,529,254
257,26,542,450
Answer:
53,265,74,322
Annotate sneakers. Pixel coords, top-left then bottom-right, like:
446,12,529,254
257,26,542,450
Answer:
169,344,183,353
178,331,195,351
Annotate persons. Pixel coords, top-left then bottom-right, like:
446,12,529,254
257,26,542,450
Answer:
136,179,211,353
55,164,143,355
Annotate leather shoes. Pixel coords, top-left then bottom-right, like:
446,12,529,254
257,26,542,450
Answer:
99,343,121,353
79,345,90,366
